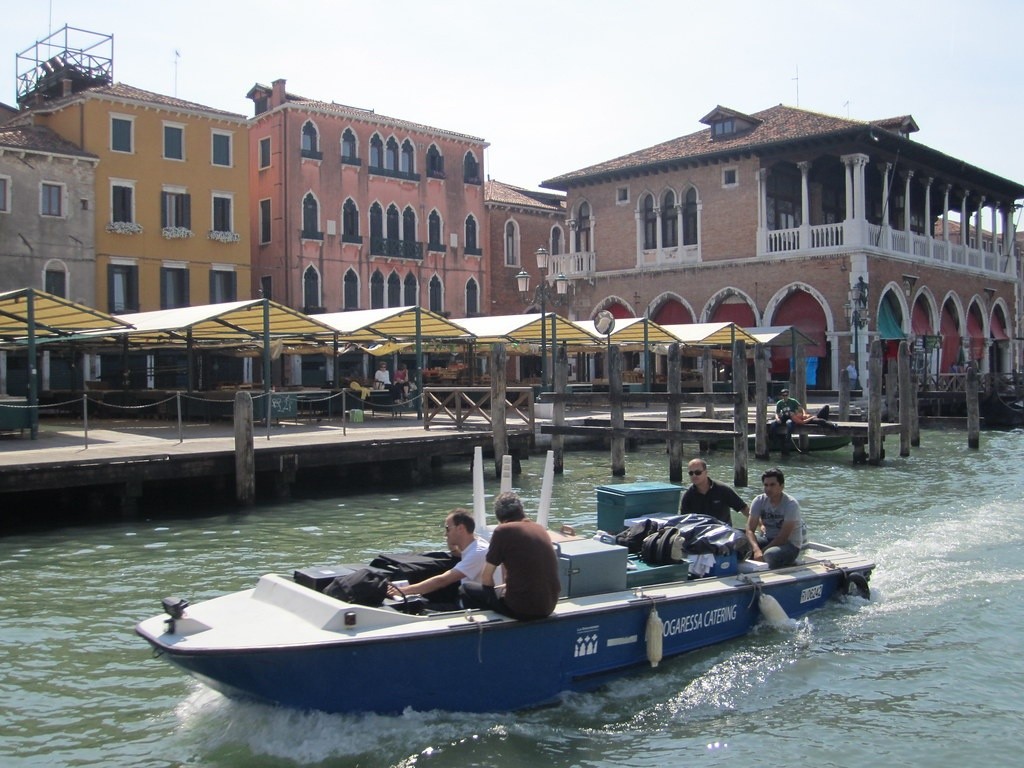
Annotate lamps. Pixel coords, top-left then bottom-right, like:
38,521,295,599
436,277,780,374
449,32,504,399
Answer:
450,234,457,248
327,220,335,235
869,131,879,140
565,219,576,231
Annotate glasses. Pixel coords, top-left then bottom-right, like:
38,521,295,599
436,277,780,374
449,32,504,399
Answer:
688,469,705,476
782,395,788,397
445,526,459,532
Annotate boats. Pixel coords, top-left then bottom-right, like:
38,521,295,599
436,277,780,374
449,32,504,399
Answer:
135,481,878,718
718,434,855,451
982,390,1024,428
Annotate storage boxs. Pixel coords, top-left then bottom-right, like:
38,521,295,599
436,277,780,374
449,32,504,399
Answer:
597,482,687,536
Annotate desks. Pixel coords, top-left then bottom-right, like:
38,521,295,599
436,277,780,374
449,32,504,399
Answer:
918,372,983,392
0,383,642,439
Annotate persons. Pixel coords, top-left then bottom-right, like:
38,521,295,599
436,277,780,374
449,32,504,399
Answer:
374,362,404,404
769,389,800,443
387,508,503,612
459,492,561,620
949,361,963,388
681,459,749,528
746,469,803,570
393,363,410,401
847,360,857,390
782,405,838,429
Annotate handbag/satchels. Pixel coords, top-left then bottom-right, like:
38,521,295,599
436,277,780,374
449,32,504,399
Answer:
617,519,664,553
643,526,685,564
